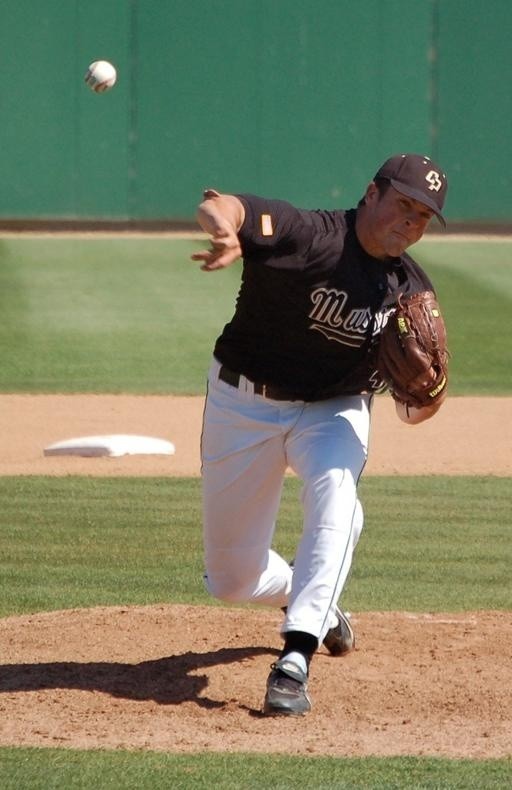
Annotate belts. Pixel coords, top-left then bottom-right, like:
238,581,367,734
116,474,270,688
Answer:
215,362,367,403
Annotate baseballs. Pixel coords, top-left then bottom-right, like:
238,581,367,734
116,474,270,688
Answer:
84,61,117,92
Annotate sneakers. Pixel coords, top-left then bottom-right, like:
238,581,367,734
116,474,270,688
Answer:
263,654,312,722
282,557,357,661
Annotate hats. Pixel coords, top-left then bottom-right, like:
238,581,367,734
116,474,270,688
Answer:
370,151,450,230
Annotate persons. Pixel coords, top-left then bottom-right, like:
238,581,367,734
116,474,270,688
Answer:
189,152,450,714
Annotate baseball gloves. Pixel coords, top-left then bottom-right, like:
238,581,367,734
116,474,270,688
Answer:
376,290,449,409
